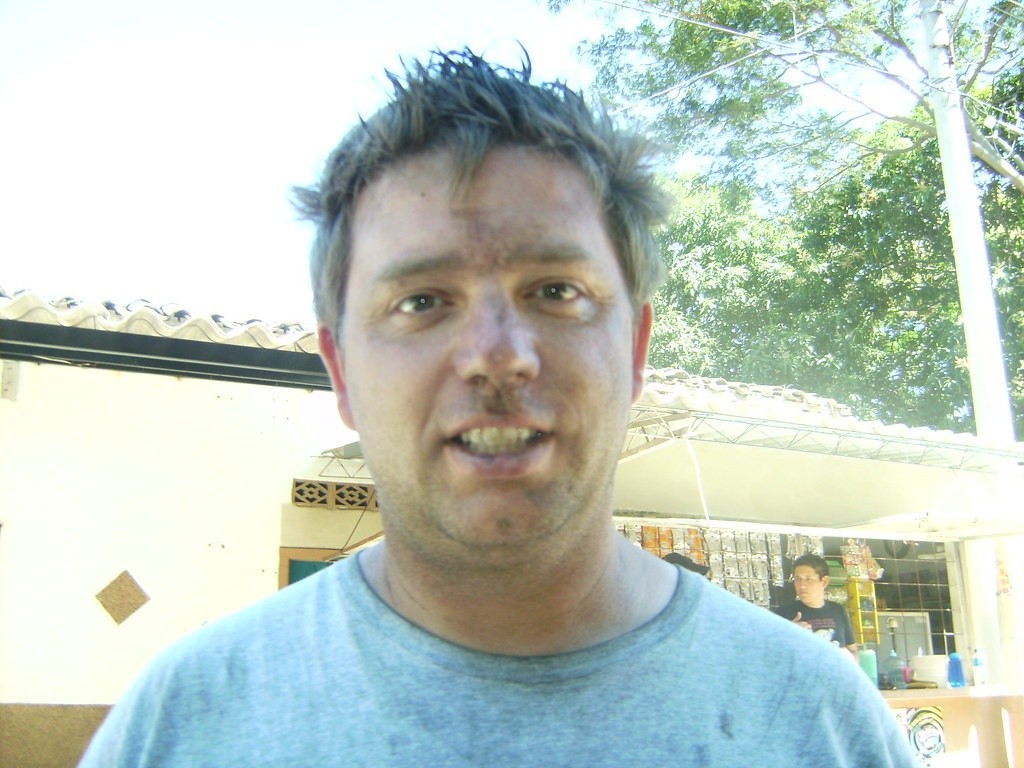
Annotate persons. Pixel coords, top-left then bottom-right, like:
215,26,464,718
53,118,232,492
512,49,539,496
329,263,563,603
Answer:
73,40,921,768
774,555,860,662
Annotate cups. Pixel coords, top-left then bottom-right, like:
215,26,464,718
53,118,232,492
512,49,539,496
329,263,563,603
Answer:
859,649,878,688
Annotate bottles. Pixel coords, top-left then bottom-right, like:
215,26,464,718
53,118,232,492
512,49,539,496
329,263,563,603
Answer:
882,650,906,688
948,647,964,687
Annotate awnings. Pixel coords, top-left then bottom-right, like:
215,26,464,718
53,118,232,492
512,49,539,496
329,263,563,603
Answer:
297,383,1024,562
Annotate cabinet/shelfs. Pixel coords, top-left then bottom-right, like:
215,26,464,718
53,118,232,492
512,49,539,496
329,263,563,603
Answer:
848,579,881,645
